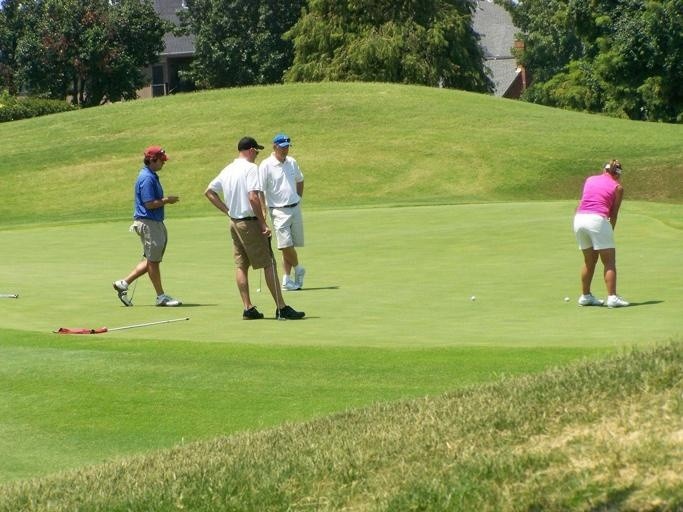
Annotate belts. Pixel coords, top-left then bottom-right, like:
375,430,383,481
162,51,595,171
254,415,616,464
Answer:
139,216,146,220
231,216,259,221
269,202,298,209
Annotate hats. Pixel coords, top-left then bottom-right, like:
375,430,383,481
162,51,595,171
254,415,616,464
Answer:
143,146,170,161
605,162,623,175
272,133,293,148
238,136,265,151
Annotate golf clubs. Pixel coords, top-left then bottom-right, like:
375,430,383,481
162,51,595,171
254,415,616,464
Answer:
268,236,285,320
0,295,18,298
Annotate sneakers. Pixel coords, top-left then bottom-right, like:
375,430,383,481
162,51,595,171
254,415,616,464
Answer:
276,305,305,320
579,295,605,305
113,280,134,306
156,296,182,307
607,295,631,307
295,267,305,288
281,281,301,291
242,309,264,320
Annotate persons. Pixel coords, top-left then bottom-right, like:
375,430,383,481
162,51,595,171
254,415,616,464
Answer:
257,131,308,292
202,135,305,322
570,157,629,310
111,146,183,309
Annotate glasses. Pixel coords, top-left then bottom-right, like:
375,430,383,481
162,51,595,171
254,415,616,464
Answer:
273,138,291,144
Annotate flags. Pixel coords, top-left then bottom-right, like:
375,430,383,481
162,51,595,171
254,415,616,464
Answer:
50,325,108,335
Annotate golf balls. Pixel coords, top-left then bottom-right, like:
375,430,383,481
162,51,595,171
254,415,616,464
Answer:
257,289,260,292
472,296,475,300
565,297,570,302
